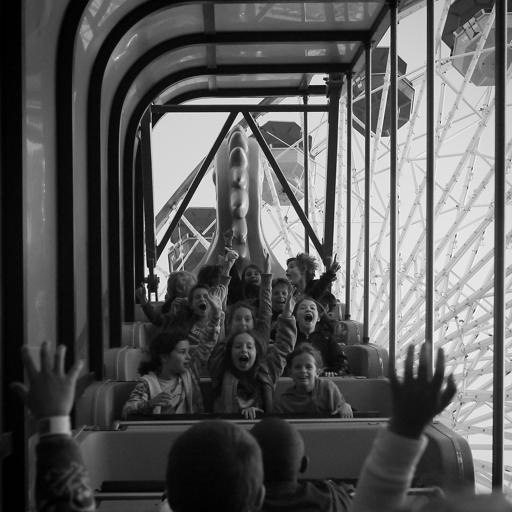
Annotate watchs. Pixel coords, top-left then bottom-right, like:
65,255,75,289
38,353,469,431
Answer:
38,415,72,438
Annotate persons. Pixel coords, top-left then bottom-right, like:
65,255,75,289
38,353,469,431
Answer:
347,342,511,509
270,253,340,326
211,302,260,359
241,342,355,420
220,250,263,310
1,341,96,512
122,289,223,419
245,419,358,509
211,286,301,417
268,299,339,348
164,420,266,512
162,283,231,338
282,254,339,299
136,272,197,325
